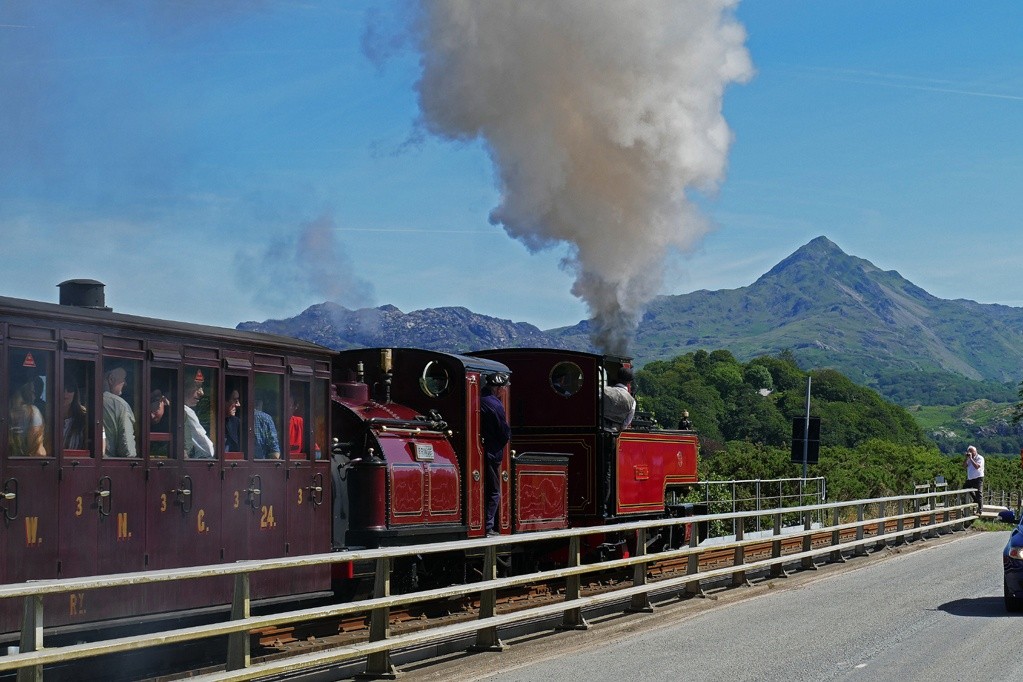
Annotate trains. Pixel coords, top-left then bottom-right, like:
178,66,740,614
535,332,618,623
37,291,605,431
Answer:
0,278,712,674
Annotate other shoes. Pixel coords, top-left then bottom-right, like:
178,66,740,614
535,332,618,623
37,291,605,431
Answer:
976,511,982,515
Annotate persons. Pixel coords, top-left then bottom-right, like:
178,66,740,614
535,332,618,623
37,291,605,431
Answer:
9,364,320,459
962,445,985,514
601,368,636,517
479,373,510,536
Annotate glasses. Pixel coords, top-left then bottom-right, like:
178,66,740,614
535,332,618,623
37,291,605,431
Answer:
231,397,240,403
64,384,75,393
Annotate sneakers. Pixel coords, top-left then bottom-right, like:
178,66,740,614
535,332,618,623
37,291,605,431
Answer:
486,529,499,535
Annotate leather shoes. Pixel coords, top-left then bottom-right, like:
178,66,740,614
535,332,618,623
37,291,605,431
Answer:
603,511,608,518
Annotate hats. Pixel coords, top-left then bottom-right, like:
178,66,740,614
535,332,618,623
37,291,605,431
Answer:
485,372,511,387
184,367,204,382
151,385,162,410
617,367,633,380
104,359,127,371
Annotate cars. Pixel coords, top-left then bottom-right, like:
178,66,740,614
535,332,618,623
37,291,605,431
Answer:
997,500,1023,612
